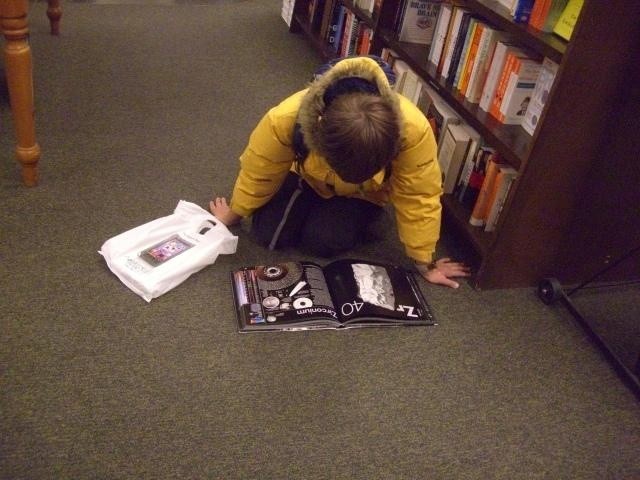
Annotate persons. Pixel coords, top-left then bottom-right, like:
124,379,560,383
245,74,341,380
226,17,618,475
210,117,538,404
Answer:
207,52,474,292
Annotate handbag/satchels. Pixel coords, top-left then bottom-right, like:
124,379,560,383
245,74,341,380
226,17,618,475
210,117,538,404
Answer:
96,199,238,303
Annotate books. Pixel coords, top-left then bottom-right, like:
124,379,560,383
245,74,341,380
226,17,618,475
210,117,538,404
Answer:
352,1,562,137
501,1,583,41
305,1,515,233
230,255,439,335
